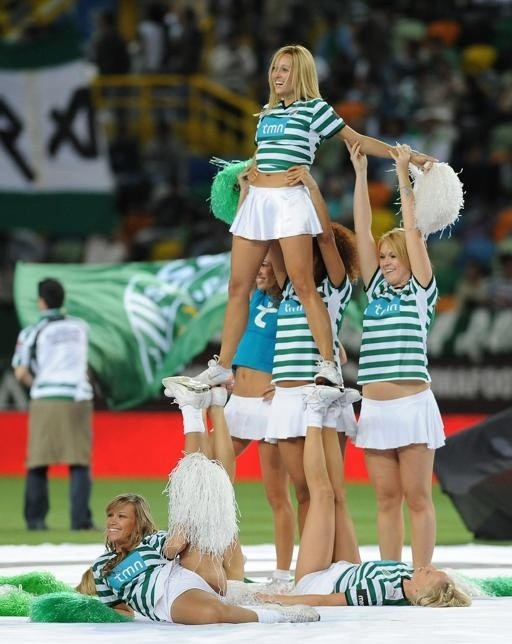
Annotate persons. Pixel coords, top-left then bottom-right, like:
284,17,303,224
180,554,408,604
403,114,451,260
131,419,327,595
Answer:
345,136,448,571
252,388,473,608
242,166,360,579
77,384,246,595
189,46,440,391
2,0,511,315
90,374,320,625
10,277,101,532
216,168,296,594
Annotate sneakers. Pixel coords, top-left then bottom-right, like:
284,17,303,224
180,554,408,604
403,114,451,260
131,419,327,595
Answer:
192,354,234,387
328,389,362,418
164,387,228,407
264,601,320,624
162,375,210,409
314,357,342,387
303,388,345,416
